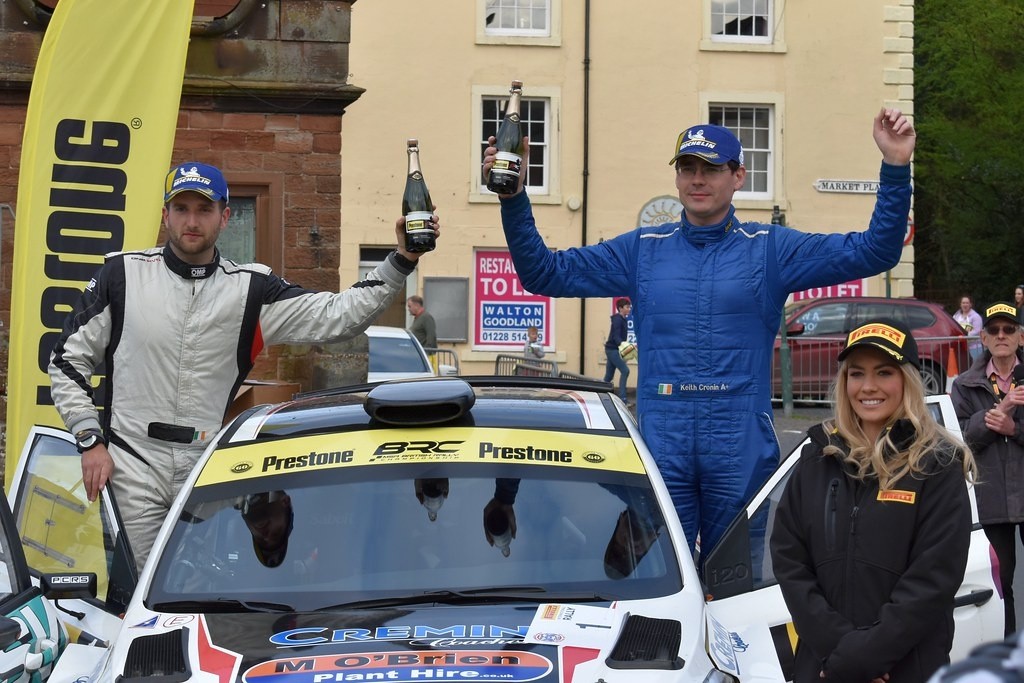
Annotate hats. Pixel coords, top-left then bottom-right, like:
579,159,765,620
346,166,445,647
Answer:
982,301,1024,330
669,124,744,168
837,318,921,374
164,163,229,206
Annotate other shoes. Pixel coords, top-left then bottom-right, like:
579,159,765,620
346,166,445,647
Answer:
623,401,636,407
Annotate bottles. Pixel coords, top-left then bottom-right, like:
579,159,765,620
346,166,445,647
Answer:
486,509,512,557
422,482,443,521
487,80,524,194
402,139,436,252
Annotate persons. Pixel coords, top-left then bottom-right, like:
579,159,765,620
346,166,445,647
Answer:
48,161,441,582
769,317,989,683
483,106,918,605
953,295,989,363
950,303,1024,643
234,479,665,582
1014,285,1024,326
512,326,546,376
603,299,637,404
404,294,440,362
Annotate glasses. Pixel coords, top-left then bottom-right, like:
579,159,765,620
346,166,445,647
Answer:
677,166,731,181
984,324,1020,335
